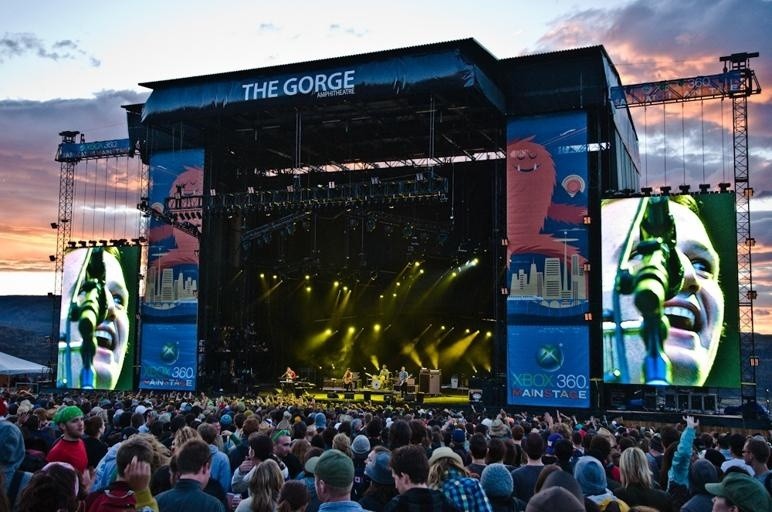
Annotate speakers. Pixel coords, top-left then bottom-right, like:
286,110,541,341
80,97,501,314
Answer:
327,393,339,398
419,374,440,395
295,387,304,398
344,394,354,399
364,391,371,400
384,394,392,400
417,393,423,402
272,388,283,395
404,394,414,400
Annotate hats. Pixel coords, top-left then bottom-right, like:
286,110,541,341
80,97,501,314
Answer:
350,435,370,453
303,449,354,486
135,405,147,415
493,420,505,432
481,462,514,496
428,447,463,466
575,456,606,494
220,415,232,425
365,452,391,484
705,472,772,511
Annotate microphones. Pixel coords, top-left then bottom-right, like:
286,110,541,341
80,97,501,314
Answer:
639,197,683,301
86,248,108,326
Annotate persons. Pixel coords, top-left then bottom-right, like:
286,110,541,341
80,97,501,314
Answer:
56,247,129,391
599,194,725,387
0,364,772,512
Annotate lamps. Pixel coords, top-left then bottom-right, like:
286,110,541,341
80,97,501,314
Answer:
499,285,509,296
49,254,59,262
743,235,756,247
744,186,754,198
583,214,591,227
605,183,737,194
583,260,591,272
501,233,509,246
161,177,450,227
51,221,60,230
67,236,146,249
748,354,759,367
47,290,54,300
584,311,594,324
745,288,758,300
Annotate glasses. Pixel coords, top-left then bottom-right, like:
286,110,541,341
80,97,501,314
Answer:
282,443,293,447
272,430,290,440
